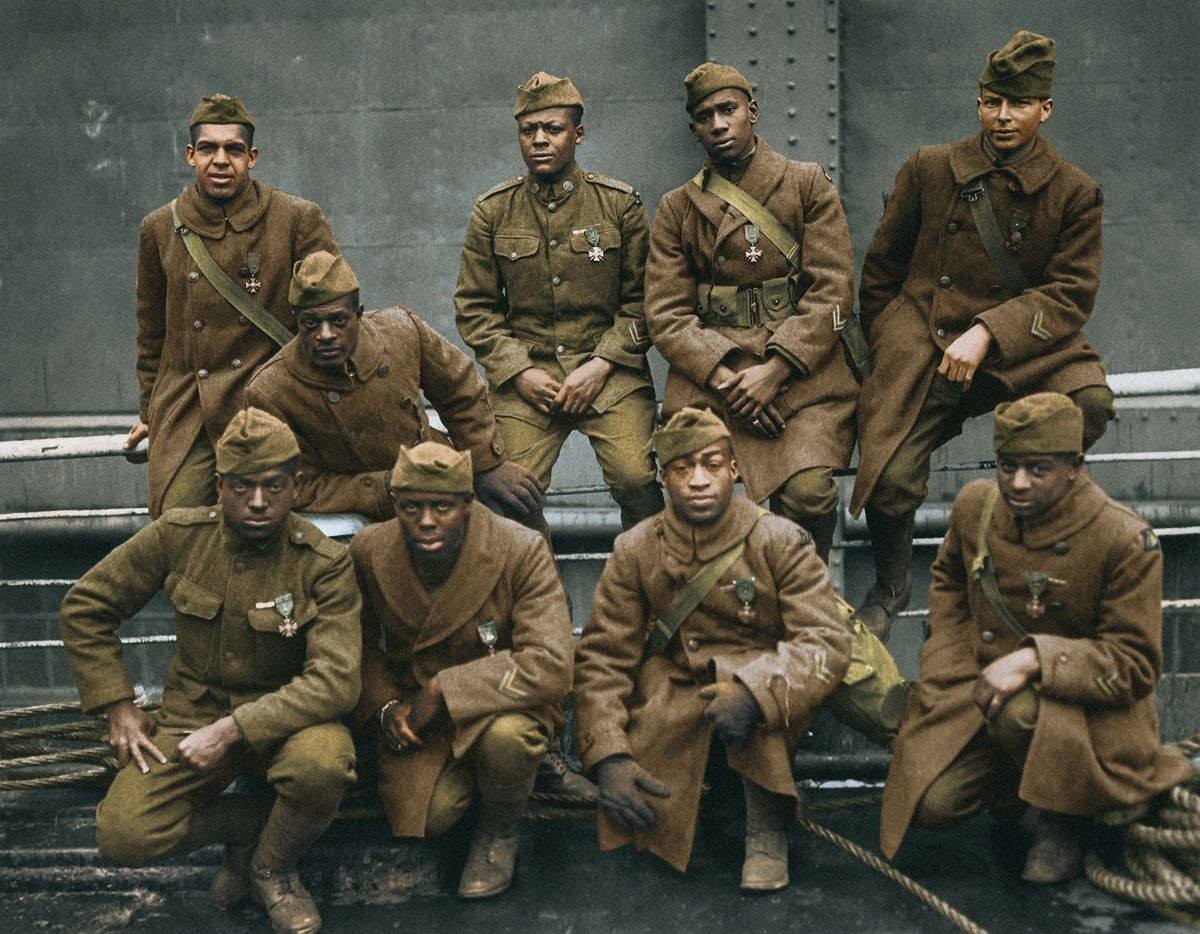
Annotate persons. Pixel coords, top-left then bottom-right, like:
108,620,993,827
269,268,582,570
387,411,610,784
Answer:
124,94,342,520
60,248,599,934
455,72,666,624
572,405,854,892
644,61,873,567
881,393,1198,891
850,31,1114,647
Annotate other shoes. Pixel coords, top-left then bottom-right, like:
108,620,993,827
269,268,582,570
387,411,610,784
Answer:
1017,814,1086,887
249,859,322,934
857,571,913,646
208,822,262,914
457,824,520,897
741,819,787,890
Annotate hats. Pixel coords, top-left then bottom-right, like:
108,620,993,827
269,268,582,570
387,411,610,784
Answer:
514,70,584,116
214,407,300,475
188,95,256,133
978,31,1056,99
685,63,753,116
650,406,729,469
993,393,1085,454
391,441,473,496
289,249,359,309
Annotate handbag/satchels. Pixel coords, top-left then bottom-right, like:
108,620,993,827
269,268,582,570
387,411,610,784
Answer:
826,589,914,753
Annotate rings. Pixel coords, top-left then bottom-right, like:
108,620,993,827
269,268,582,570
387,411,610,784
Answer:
991,698,1003,709
752,419,759,427
397,744,401,751
